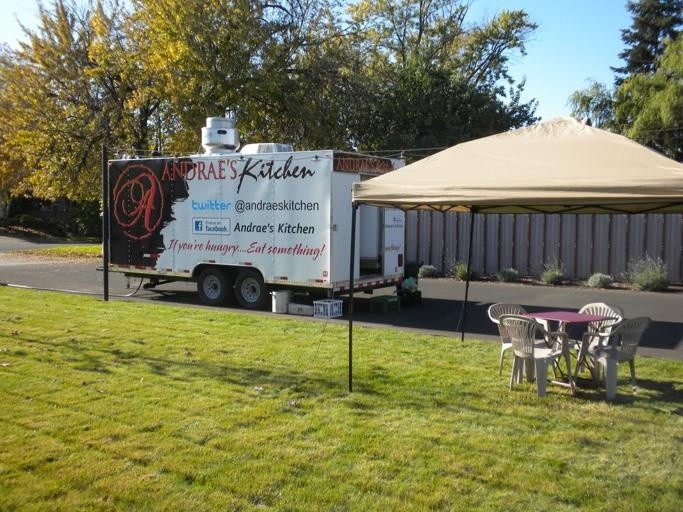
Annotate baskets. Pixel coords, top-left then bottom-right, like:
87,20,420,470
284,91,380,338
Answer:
313,298,342,318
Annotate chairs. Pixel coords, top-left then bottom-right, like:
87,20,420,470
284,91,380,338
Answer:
484,302,653,402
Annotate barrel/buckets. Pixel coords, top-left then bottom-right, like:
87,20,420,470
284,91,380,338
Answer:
270,290,290,314
